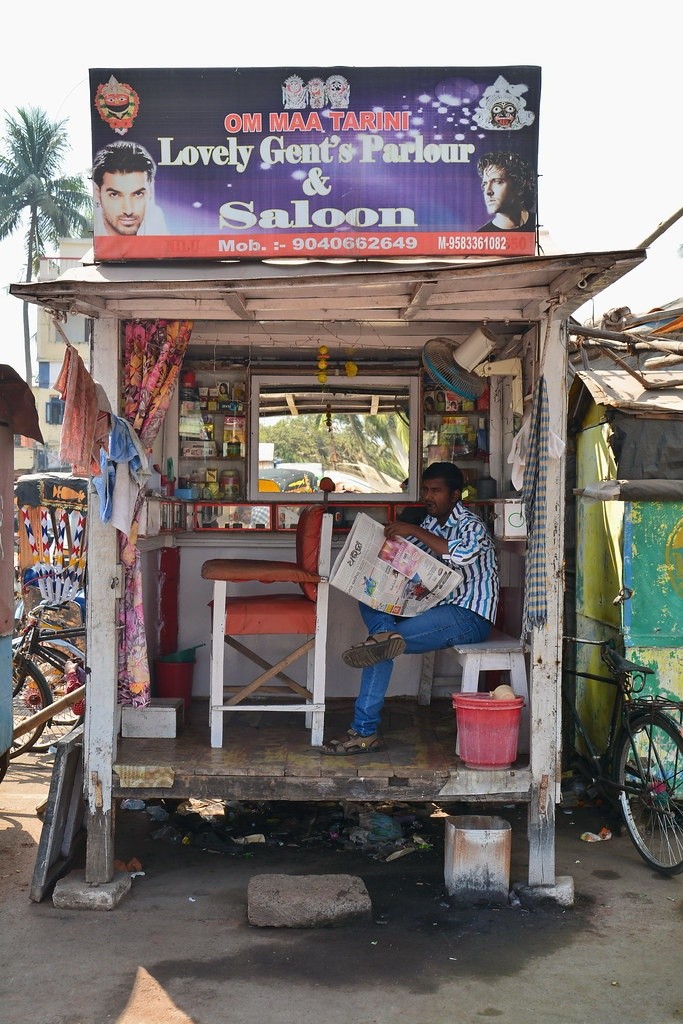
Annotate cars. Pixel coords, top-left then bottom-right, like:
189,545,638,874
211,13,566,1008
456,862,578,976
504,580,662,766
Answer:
259,464,379,494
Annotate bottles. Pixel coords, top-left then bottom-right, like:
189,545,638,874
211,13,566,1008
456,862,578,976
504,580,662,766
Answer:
209,483,219,499
227,441,241,459
221,470,240,500
190,470,199,483
192,484,201,499
202,482,211,500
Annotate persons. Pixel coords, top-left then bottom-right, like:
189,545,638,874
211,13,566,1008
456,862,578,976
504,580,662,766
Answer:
93,140,162,236
425,395,434,411
220,383,228,396
435,391,447,411
474,151,535,233
318,461,501,761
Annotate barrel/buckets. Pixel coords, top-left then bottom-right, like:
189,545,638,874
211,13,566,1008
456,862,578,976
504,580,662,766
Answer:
445,815,512,908
154,660,197,713
451,691,526,771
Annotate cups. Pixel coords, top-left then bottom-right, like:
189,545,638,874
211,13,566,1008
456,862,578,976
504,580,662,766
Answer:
490,684,517,700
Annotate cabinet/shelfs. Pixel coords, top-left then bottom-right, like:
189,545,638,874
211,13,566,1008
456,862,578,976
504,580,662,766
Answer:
176,365,246,503
420,367,490,498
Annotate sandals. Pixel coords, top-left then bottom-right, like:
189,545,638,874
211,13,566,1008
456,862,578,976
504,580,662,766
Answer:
320,728,384,755
342,633,406,668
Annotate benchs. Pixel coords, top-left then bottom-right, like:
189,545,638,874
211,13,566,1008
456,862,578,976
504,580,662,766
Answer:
415,624,531,756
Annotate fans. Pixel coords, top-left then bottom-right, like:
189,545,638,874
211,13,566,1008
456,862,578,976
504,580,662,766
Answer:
421,327,523,419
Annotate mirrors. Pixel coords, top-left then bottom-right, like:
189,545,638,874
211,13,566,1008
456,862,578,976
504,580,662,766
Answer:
251,376,415,501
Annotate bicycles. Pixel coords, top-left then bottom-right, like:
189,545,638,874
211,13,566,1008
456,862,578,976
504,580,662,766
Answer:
9,602,86,759
561,633,683,875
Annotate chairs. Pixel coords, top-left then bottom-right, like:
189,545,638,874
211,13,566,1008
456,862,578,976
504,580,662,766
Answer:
200,506,334,748
